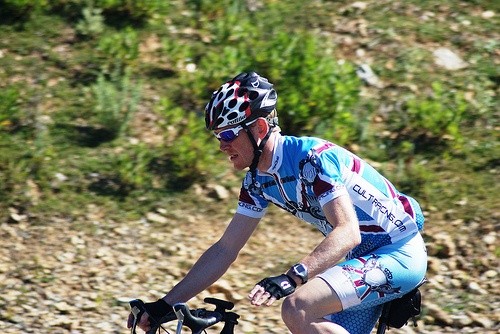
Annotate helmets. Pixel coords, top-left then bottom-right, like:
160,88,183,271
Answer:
204,72,277,130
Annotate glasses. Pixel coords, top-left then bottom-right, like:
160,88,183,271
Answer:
213,117,273,143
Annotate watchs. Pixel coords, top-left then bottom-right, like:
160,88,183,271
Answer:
291,262,308,284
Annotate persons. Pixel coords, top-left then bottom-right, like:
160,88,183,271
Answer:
128,72,427,334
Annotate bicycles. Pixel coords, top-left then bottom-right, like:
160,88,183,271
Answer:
127,288,422,334
